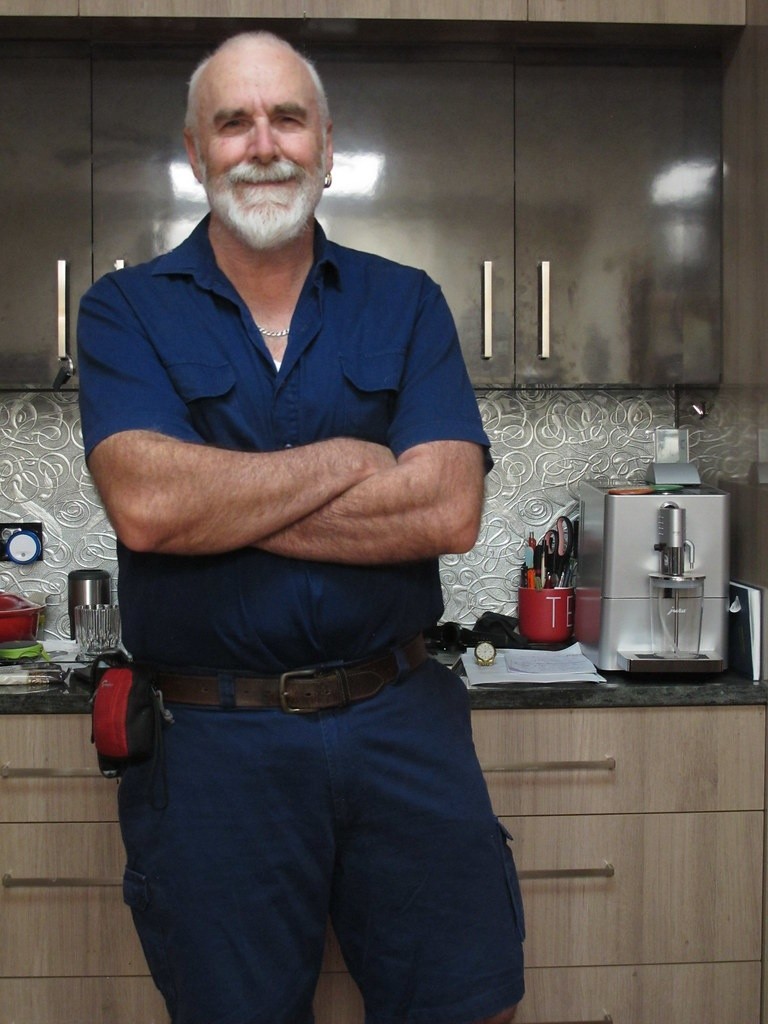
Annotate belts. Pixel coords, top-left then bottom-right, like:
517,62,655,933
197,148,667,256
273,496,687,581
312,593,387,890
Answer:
155,633,429,714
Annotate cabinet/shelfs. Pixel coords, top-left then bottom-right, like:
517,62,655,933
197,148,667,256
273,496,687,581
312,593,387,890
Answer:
0,707,768,1024
0,38,724,392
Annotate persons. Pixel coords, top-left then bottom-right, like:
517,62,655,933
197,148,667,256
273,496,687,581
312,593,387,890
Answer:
73,32,534,1024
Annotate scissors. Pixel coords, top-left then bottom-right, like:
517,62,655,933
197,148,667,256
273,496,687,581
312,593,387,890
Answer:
543,516,573,579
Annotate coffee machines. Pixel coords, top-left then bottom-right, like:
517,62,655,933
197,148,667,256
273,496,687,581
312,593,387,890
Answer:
578,479,734,676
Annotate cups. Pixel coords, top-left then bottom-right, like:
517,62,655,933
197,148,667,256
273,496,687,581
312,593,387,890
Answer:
75,604,121,654
519,586,574,643
649,572,704,659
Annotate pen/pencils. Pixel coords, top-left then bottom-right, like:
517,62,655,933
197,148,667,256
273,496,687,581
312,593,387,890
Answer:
520,520,579,591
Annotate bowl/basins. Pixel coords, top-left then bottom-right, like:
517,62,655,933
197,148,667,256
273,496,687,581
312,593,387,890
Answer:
0,593,43,639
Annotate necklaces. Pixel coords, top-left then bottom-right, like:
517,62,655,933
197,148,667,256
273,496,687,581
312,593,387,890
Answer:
256,322,291,339
